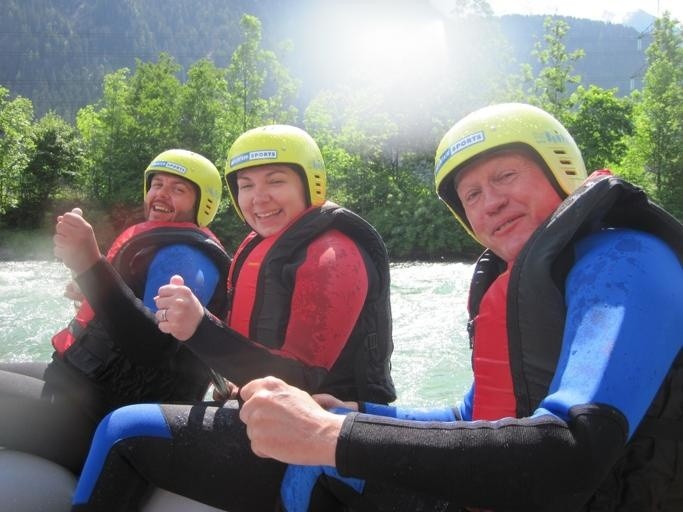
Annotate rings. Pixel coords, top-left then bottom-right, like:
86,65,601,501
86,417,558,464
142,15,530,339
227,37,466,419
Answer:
159,309,168,323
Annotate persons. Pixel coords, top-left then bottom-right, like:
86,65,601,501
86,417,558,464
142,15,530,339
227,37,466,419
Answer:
236,100,682,512
69,122,395,510
0,147,233,483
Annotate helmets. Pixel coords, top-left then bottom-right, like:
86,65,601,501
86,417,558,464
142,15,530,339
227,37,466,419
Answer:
223,123,326,224
434,101,588,247
141,148,221,227
